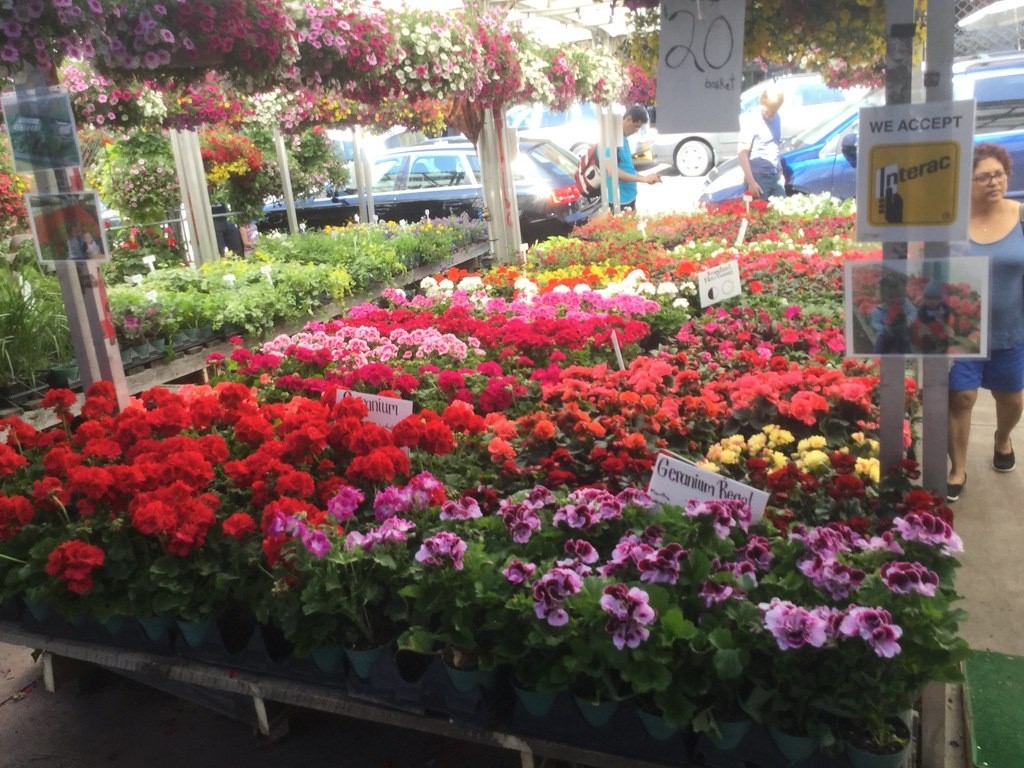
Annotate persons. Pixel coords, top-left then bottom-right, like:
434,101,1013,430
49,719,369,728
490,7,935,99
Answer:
67,226,105,260
738,87,786,202
202,154,244,258
948,141,1024,501
597,105,662,215
240,220,259,258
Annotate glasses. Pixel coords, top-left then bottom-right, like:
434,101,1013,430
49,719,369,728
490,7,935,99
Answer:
972,171,1006,183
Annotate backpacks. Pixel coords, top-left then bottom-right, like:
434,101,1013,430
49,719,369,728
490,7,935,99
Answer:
575,144,604,199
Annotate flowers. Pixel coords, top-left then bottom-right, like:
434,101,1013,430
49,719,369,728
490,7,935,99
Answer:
0,190,974,729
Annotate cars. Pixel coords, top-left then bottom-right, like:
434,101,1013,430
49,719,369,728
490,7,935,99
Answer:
696,57,1024,220
651,71,886,178
257,142,591,245
359,102,649,191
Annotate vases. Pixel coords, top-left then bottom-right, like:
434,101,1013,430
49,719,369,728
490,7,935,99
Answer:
258,614,298,664
0,379,28,406
49,368,68,387
341,620,398,682
767,718,821,764
172,329,184,345
200,323,212,338
56,596,89,627
847,716,911,768
120,347,131,362
573,686,619,728
702,708,755,750
635,699,682,741
52,363,78,381
508,667,561,717
308,628,347,676
174,611,208,648
137,609,171,641
22,594,49,621
214,603,257,654
441,647,497,697
183,327,199,341
99,612,127,634
132,340,149,356
393,646,434,687
151,337,165,351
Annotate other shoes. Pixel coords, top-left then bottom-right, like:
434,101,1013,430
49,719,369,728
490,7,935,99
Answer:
992,429,1017,472
946,464,967,500
